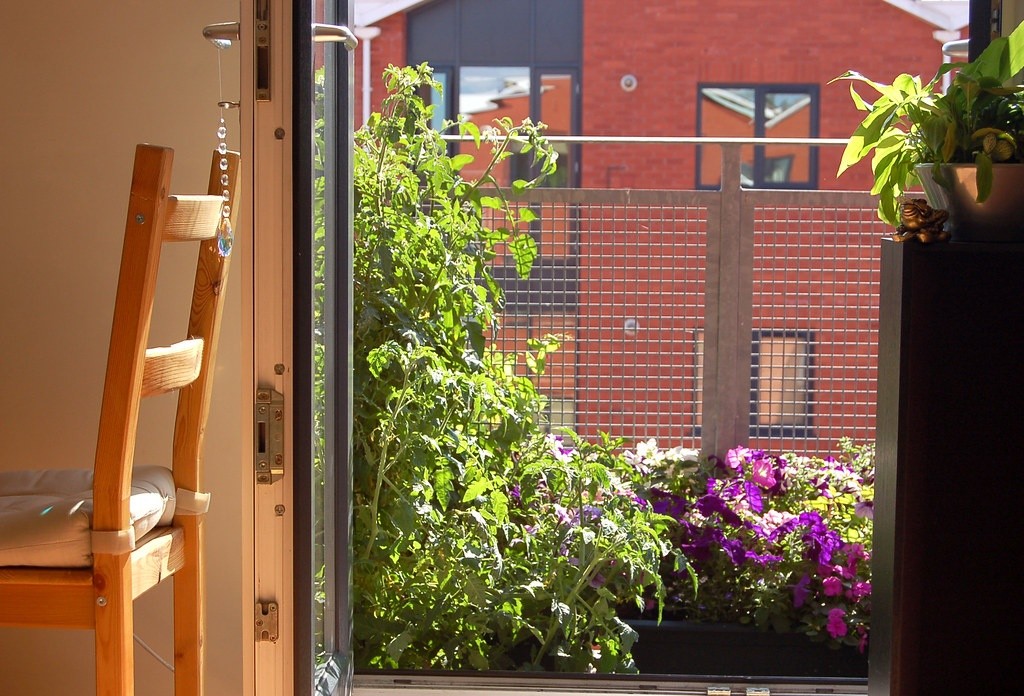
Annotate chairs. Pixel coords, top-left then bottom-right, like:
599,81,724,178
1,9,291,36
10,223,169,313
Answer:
0,141,242,696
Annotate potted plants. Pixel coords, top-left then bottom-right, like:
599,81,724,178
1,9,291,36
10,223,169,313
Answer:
827,20,1024,243
